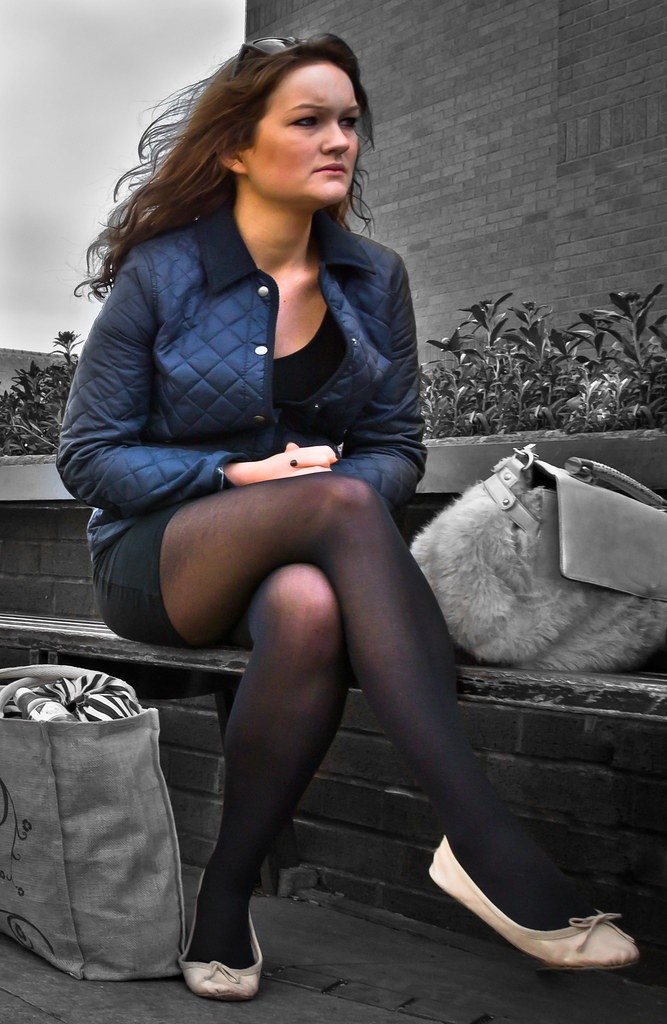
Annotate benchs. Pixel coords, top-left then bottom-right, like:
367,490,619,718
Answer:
0,612,667,893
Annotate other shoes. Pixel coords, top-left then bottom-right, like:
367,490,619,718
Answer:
428,836,641,971
178,868,261,1000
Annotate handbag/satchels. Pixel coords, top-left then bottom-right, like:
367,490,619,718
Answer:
0,665,186,981
411,442,667,679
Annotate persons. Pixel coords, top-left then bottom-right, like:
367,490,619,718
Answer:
58,31,640,1001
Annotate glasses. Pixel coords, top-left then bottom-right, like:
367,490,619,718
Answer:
231,37,310,79
